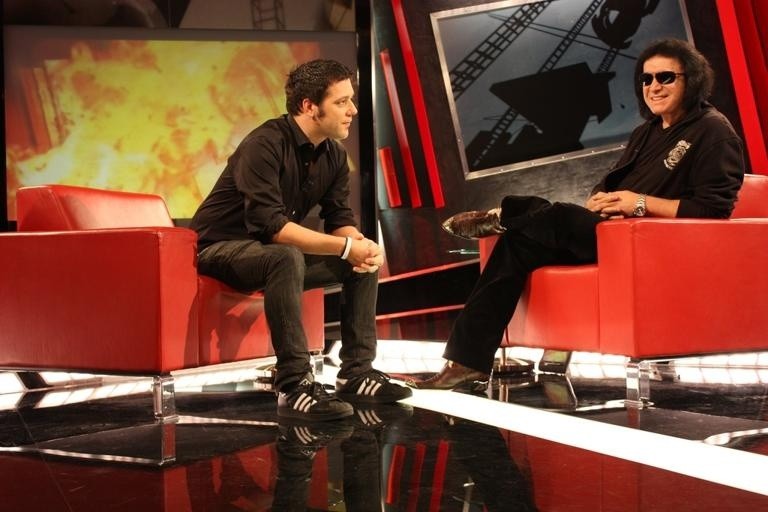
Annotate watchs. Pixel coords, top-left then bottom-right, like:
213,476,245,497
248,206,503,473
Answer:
631,192,648,218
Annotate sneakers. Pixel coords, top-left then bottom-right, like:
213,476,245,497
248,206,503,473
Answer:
275,380,356,422
273,419,355,460
348,398,415,434
336,367,412,403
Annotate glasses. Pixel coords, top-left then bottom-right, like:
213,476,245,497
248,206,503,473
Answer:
638,70,687,86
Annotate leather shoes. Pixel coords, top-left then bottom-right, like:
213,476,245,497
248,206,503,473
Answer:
407,358,493,391
443,205,508,240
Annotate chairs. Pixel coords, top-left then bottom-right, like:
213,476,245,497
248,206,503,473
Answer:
3,183,328,430
476,169,767,406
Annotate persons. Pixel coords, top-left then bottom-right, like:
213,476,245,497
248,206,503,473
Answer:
401,36,746,391
426,392,543,512
268,403,414,512
186,52,413,422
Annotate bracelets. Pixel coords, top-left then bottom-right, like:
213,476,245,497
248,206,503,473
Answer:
338,236,353,261
